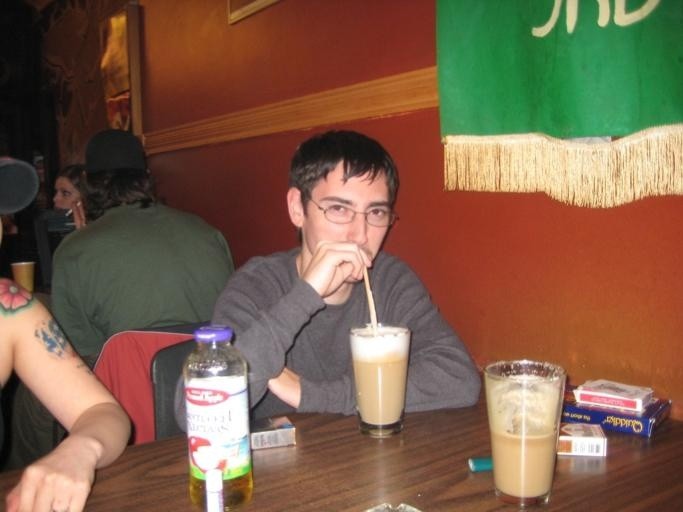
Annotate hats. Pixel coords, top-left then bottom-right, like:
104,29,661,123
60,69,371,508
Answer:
86,129,146,170
0,156,39,215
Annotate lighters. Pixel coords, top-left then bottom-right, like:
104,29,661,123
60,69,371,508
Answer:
467,456,494,473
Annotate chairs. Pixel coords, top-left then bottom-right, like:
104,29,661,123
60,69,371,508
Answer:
149,340,205,439
82,319,222,445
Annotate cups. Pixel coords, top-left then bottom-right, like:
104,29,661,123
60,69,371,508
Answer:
348,435,411,512
347,323,412,436
10,261,35,294
480,358,567,505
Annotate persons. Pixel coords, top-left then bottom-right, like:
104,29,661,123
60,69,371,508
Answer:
170,128,481,433
49,164,90,250
0,156,133,512
7,128,235,470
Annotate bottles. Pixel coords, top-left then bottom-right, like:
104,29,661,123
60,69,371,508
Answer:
179,324,256,508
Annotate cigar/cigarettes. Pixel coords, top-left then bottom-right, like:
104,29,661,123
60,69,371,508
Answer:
63,202,81,218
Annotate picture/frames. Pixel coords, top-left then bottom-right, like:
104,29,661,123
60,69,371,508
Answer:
225,0,280,25
33,0,143,209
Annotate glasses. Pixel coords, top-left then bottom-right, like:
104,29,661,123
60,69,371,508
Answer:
306,189,399,227
